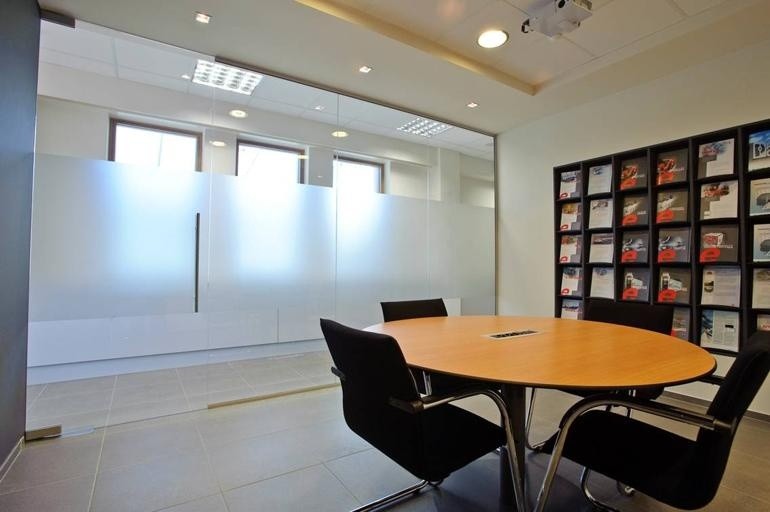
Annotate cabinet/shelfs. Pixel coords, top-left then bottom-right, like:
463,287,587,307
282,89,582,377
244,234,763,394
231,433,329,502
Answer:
553,118,770,359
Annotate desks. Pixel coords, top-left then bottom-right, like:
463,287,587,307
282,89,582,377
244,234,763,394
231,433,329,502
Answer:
365,318,716,512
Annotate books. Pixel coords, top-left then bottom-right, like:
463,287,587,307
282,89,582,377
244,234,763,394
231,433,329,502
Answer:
587,165,615,300
558,169,585,321
654,150,691,340
618,158,650,302
695,132,769,353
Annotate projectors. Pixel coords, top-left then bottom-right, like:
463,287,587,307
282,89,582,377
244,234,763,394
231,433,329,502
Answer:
528,0,593,37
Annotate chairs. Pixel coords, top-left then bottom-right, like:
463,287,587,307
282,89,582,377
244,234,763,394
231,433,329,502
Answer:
528,296,675,451
317,316,526,512
380,299,507,456
526,331,770,512
